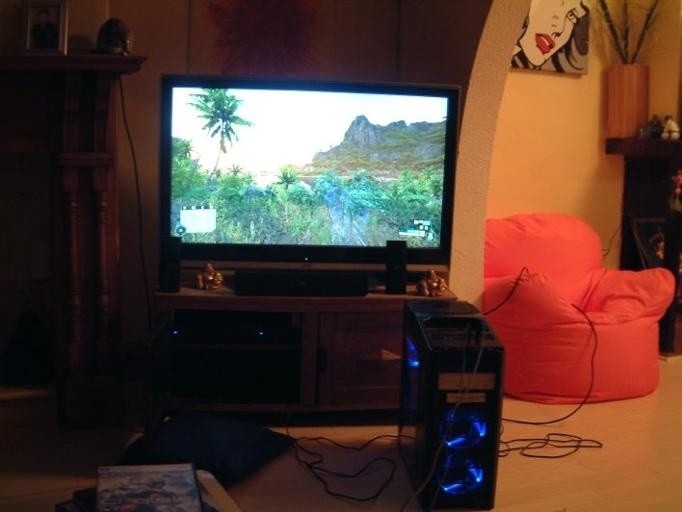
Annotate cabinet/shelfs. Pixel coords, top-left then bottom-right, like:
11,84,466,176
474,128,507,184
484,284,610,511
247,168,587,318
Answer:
147,275,457,414
607,136,682,355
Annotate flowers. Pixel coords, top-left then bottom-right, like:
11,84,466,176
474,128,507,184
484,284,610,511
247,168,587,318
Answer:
598,0,658,63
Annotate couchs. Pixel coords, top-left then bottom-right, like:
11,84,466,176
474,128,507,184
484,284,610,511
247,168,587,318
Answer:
479,214,674,403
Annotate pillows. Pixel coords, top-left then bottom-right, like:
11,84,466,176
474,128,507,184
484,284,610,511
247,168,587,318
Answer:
120,412,299,487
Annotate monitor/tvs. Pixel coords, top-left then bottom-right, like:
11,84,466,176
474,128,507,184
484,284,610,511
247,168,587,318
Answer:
158,72,464,299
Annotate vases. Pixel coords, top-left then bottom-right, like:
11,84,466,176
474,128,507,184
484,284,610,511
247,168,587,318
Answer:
606,62,647,137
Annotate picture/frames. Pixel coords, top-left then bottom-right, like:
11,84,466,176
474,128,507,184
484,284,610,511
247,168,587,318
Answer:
509,0,591,74
27,1,68,56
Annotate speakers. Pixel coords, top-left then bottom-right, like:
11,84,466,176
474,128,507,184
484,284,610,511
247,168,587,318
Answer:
385,242,407,294
158,237,182,294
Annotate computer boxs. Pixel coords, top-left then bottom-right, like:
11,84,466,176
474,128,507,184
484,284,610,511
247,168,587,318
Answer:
396,300,505,512
